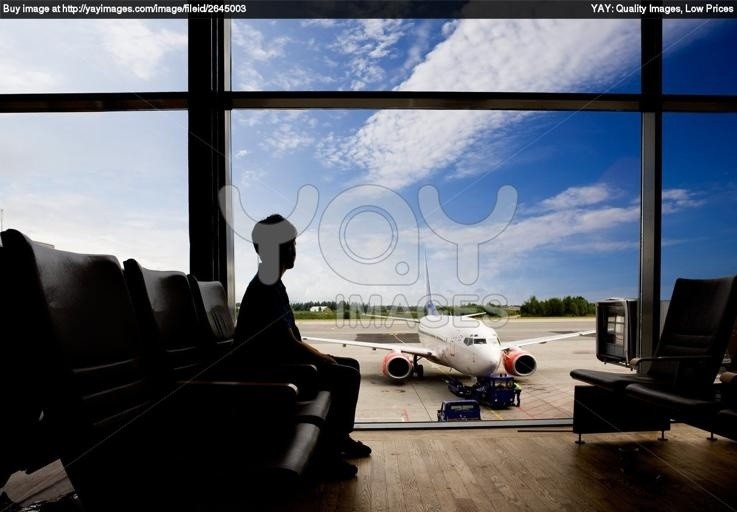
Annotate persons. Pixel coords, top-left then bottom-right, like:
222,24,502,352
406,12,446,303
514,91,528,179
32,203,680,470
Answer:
231,213,371,484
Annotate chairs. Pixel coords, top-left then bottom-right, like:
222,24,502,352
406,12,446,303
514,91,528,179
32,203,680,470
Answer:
570,278,736,445
0,228,332,512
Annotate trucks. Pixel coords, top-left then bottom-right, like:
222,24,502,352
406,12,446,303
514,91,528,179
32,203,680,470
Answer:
436,397,486,422
444,372,520,408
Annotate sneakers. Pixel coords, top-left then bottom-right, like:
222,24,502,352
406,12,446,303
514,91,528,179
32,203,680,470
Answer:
306,457,357,482
334,434,372,459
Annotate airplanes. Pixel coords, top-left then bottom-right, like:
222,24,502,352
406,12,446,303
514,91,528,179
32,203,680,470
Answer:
302,244,614,376
